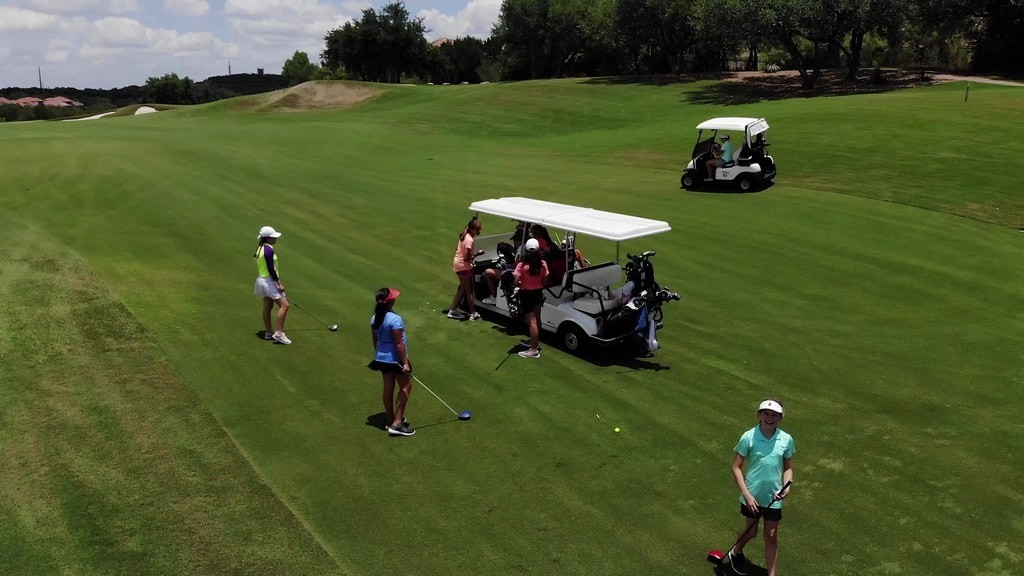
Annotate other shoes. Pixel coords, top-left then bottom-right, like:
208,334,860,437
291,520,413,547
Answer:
704,178,714,181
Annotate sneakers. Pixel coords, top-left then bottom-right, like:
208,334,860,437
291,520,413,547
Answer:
729,549,748,575
482,295,497,304
519,339,539,348
387,416,407,429
447,309,465,318
470,312,480,321
518,348,540,358
264,331,275,339
272,330,292,345
388,424,416,435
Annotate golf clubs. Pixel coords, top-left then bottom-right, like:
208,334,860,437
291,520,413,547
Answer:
282,291,338,331
706,479,794,563
397,362,472,419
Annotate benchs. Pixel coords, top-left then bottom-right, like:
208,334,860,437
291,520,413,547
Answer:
572,263,628,316
726,138,765,167
542,261,614,305
901,66,936,81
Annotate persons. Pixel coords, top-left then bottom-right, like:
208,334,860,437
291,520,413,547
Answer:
530,224,553,260
254,226,292,345
447,219,485,321
482,231,529,304
513,238,549,358
367,288,415,436
704,133,734,182
728,398,796,576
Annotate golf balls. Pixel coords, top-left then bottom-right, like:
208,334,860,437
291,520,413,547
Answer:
614,427,620,433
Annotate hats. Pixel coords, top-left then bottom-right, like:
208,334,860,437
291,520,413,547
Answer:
383,288,400,302
510,234,520,240
719,134,729,138
758,399,784,416
526,237,539,251
258,226,281,240
